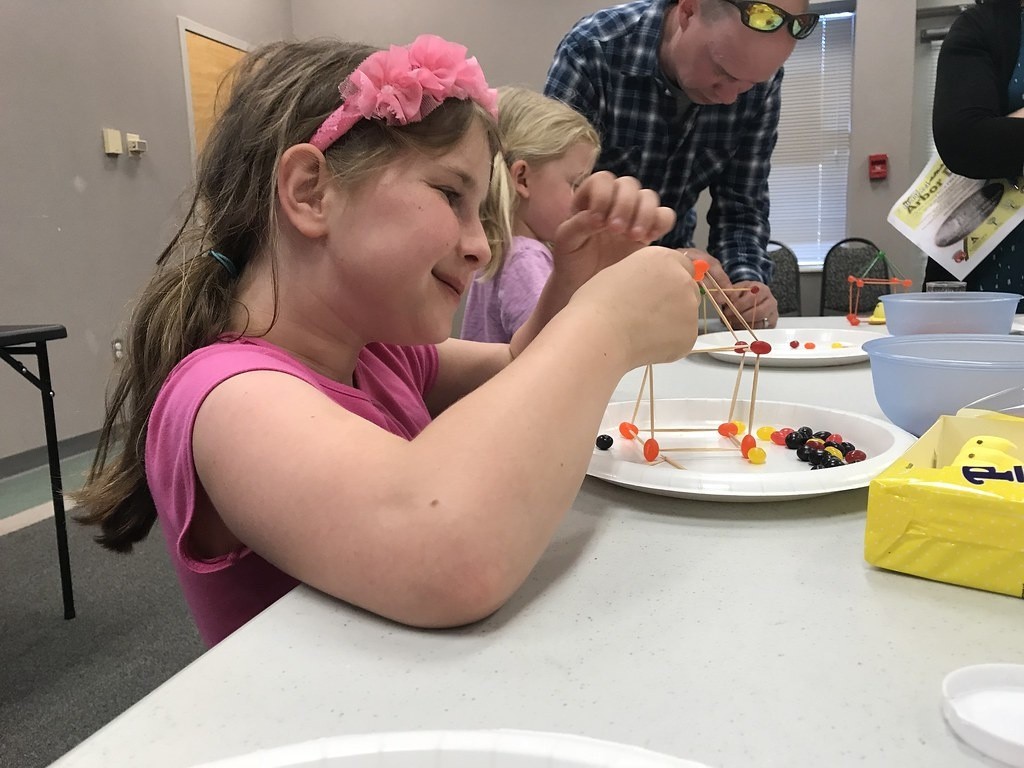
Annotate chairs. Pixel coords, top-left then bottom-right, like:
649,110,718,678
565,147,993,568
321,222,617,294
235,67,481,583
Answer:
820,238,888,314
764,239,802,318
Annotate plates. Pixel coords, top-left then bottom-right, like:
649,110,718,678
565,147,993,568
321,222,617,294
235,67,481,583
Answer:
587,398,918,503
926,281,968,293
693,328,883,367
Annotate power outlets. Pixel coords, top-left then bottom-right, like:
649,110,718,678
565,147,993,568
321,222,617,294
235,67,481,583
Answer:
111,335,125,362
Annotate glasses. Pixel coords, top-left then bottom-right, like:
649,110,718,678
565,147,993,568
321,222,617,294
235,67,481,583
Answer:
725,0,820,39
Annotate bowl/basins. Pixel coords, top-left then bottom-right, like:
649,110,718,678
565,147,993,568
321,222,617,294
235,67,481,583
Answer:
861,333,1024,437
878,292,1024,337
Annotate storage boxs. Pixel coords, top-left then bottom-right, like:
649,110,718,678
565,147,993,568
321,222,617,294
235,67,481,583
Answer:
863,411,1024,600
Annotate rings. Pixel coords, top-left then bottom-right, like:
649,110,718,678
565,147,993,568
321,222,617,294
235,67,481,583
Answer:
684,250,688,256
764,318,769,329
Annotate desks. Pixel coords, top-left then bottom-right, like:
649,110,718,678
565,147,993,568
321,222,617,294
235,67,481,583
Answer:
29,312,1024,768
0,323,77,626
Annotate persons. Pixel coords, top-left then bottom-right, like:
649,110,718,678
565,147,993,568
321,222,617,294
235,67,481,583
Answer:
921,0,1024,314
543,0,809,330
459,83,602,343
56,32,701,651
